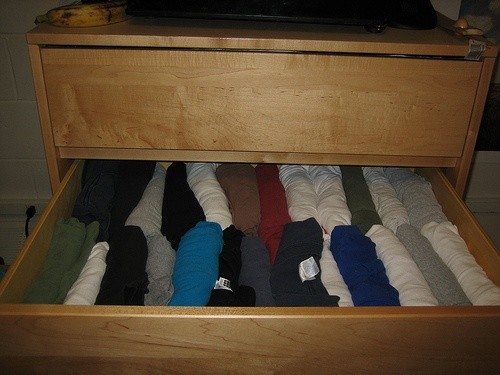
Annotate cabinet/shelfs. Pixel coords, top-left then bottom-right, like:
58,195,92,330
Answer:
0,10,500,375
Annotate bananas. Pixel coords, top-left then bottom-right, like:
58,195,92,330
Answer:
34,0,128,27
